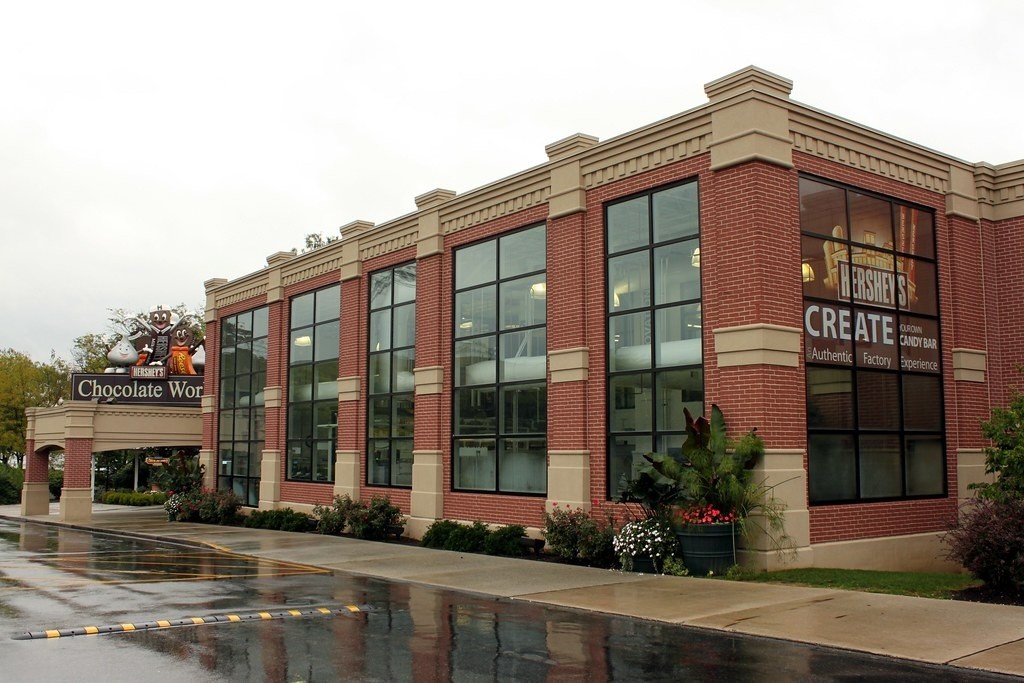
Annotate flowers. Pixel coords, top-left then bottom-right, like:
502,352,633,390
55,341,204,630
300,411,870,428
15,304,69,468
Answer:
611,516,676,562
315,494,408,538
537,500,608,558
640,402,802,561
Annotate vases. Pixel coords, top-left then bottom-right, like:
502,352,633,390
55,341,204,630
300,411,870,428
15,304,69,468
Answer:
679,521,739,574
632,556,662,573
550,551,608,567
366,524,404,541
